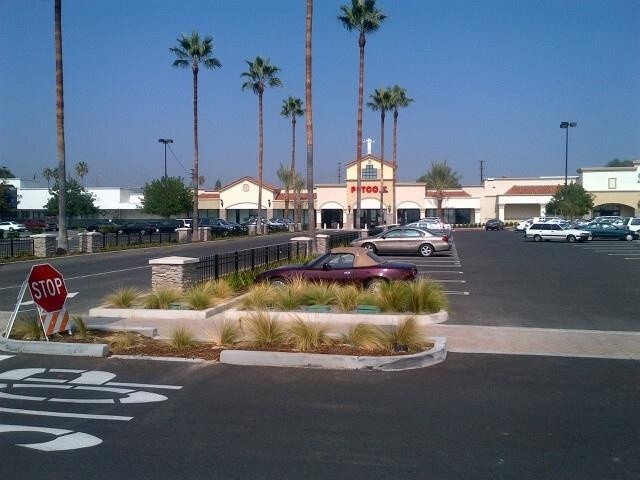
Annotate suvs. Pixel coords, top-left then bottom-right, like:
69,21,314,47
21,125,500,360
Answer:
25,219,58,231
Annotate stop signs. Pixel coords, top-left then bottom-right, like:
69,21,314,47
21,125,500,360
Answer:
28,264,71,313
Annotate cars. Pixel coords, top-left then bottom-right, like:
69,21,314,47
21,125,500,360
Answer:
407,217,452,239
349,226,452,257
87,216,294,239
486,218,505,231
513,216,640,243
0,221,26,232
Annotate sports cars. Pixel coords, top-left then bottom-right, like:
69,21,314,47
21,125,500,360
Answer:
254,246,420,294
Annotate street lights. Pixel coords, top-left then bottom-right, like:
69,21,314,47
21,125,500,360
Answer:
157,138,174,180
560,121,577,186
